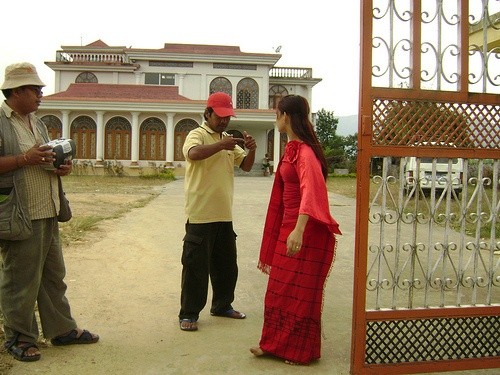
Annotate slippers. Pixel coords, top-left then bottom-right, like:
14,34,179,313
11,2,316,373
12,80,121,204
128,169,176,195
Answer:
179,318,198,331
210,308,247,319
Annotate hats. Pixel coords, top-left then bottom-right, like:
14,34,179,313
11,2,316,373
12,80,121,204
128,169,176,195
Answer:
0,61,46,89
207,91,237,117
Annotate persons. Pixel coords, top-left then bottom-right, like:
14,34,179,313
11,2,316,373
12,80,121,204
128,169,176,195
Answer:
250,94,342,366
0,62,99,361
261,154,272,176
179,92,257,330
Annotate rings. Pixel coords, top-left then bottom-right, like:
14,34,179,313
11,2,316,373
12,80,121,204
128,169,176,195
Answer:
296,247,298,248
42,157,45,161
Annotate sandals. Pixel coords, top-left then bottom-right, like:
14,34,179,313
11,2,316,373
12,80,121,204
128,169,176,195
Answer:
5,341,41,363
51,329,99,347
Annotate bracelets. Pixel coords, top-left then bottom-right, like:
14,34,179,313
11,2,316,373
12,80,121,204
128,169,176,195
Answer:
23,154,28,164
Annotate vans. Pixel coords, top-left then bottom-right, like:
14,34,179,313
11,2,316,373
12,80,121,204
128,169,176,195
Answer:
400,141,464,198
